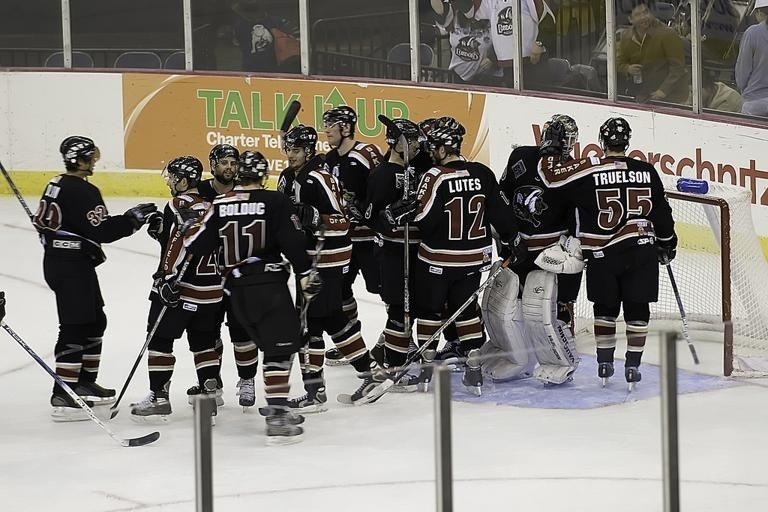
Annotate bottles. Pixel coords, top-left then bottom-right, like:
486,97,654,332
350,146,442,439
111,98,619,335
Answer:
633,66,644,86
675,178,709,195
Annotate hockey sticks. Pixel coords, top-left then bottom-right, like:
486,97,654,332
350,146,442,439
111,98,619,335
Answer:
278,101,303,156
0,321,159,448
378,115,411,336
336,262,511,406
92,251,192,419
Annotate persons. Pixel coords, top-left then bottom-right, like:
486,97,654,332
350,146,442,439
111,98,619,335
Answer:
129,155,223,425
32,136,158,420
187,143,259,413
277,105,678,445
212,150,312,445
232,0,768,118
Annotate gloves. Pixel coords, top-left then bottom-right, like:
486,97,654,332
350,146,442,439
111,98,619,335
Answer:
655,233,680,265
127,197,155,228
385,199,418,227
152,271,182,307
534,233,587,278
500,234,527,265
540,124,564,158
296,201,320,230
293,268,323,298
147,211,165,240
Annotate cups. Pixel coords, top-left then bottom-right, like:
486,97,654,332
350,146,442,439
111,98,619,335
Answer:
530,40,543,64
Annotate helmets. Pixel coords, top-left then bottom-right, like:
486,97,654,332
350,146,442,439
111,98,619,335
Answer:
166,155,204,192
417,115,437,138
285,124,318,161
599,118,630,147
207,145,237,180
239,151,269,179
322,104,358,140
60,136,100,172
539,113,580,150
384,119,420,153
428,117,464,156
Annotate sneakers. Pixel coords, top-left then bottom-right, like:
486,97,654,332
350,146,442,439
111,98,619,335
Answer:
76,379,118,401
127,382,175,418
235,335,482,408
48,391,94,411
186,373,223,395
623,366,643,382
268,423,304,437
193,398,219,417
265,410,305,425
595,362,614,376
